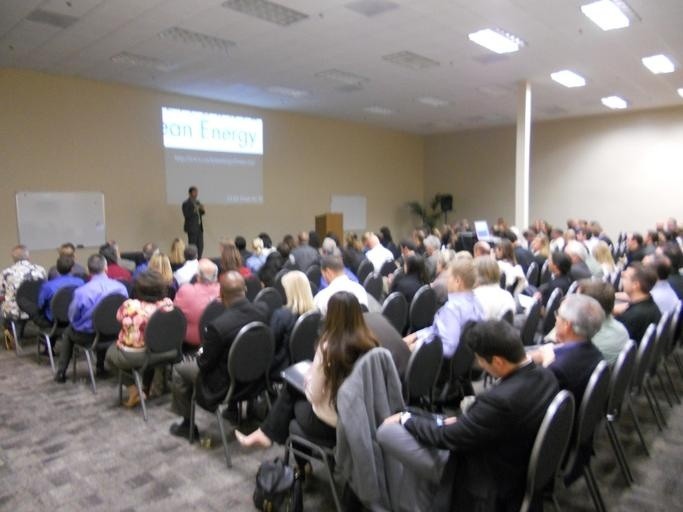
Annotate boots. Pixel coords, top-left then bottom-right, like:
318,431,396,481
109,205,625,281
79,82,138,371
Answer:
122,384,147,407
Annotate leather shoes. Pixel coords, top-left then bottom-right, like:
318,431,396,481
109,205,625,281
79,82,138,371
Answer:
235,429,272,447
96,368,104,376
41,348,55,356
171,423,198,439
55,371,66,382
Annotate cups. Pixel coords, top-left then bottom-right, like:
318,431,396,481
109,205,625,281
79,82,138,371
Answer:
198,431,211,448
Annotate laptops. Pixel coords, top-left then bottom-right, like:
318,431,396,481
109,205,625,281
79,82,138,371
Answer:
474,219,500,243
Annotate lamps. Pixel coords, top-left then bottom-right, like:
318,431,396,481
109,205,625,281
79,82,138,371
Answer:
468,0,683,109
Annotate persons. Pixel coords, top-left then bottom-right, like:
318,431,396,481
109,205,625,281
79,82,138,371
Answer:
182,186,205,261
1,220,683,512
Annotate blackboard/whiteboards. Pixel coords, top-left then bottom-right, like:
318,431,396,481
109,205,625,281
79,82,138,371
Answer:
16,190,106,251
331,194,369,232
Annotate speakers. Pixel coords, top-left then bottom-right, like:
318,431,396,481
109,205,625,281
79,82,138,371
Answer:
441,195,452,211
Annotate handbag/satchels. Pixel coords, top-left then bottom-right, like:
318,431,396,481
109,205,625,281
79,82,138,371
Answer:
254,457,303,512
1,326,14,350
